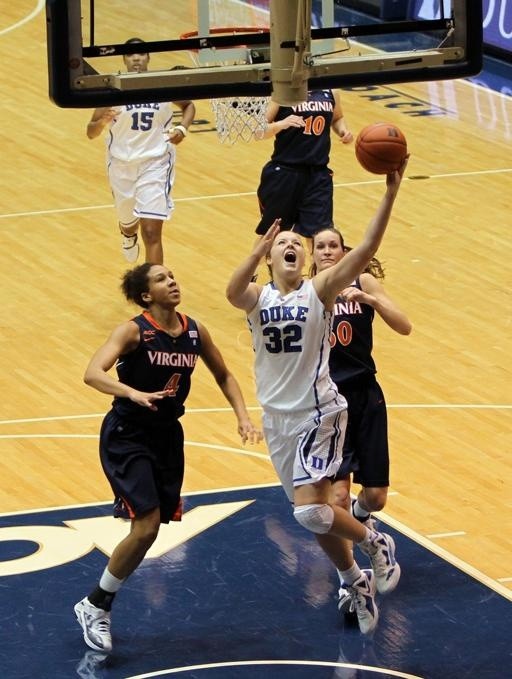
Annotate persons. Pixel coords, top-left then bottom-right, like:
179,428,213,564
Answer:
71,261,263,655
306,225,411,615
84,39,196,268
224,151,412,637
254,88,354,241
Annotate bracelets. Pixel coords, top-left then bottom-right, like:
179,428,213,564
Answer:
174,124,188,137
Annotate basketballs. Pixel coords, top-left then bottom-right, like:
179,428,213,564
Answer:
354,122,404,174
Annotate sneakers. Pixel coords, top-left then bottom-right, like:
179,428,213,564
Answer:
75,596,115,651
336,586,352,612
118,226,143,264
349,500,373,556
352,569,379,634
362,533,402,595
